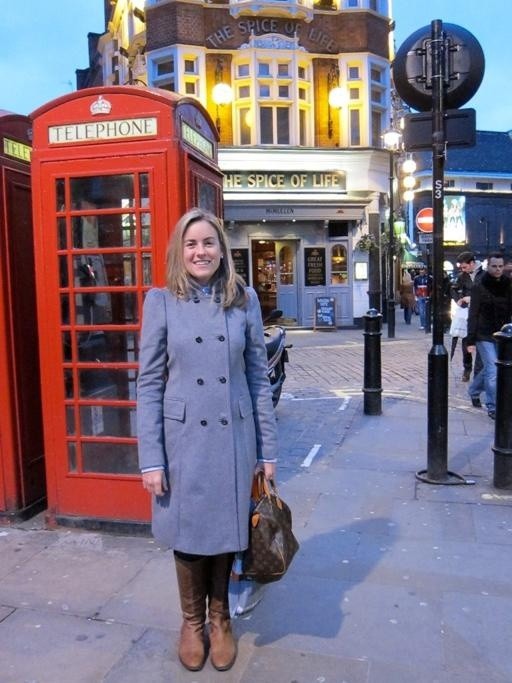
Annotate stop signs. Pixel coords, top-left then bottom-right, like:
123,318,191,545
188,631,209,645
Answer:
415,206,434,234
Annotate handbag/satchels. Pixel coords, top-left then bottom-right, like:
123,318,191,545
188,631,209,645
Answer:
449,298,469,338
239,471,300,583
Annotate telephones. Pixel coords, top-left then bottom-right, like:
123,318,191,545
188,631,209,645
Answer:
79,264,97,307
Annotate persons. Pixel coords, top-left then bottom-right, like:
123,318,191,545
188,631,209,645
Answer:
134,205,277,672
400,251,512,420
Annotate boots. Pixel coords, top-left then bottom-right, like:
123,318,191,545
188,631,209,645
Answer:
173,549,210,671
207,551,236,671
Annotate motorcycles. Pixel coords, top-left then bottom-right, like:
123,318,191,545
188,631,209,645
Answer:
248,282,294,414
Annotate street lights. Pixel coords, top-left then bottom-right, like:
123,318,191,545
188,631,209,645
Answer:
379,122,404,338
479,215,491,253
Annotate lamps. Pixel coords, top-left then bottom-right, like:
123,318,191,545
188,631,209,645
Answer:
212,59,234,134
327,63,350,139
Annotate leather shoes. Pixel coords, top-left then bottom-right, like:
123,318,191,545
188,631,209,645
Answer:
472,398,481,407
488,412,496,420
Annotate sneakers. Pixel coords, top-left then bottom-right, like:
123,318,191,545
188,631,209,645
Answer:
462,367,472,382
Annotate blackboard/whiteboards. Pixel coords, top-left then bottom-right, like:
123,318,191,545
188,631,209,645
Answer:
313,296,337,328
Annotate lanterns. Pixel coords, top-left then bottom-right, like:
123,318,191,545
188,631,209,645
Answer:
212,82,235,106
403,159,417,202
327,85,351,109
